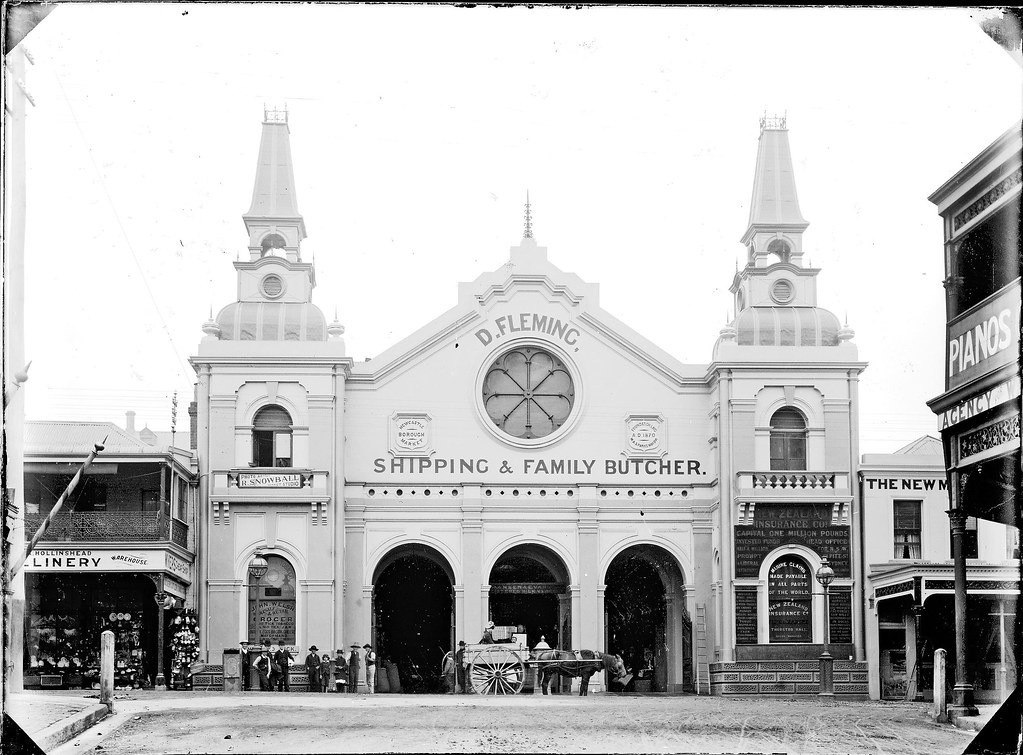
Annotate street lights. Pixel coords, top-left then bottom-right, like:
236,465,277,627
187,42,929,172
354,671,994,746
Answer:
816,555,837,702
248,548,266,691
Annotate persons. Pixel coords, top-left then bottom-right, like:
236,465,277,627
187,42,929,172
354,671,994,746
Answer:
345,642,361,693
455,641,467,694
334,650,348,693
442,653,455,695
305,645,321,692
319,654,332,693
163,643,176,691
479,621,495,644
239,641,251,690
253,638,282,692
363,644,376,694
274,640,295,692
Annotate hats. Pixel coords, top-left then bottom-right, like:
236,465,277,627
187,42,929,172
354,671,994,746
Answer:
264,638,272,646
309,646,319,651
350,644,361,648
458,641,466,646
278,640,286,645
336,650,344,653
260,645,268,652
239,642,251,645
363,644,372,649
486,621,494,628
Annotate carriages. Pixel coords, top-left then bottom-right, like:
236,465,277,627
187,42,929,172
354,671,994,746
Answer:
463,633,626,696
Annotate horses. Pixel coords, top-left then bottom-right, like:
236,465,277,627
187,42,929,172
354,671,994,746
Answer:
536,649,628,697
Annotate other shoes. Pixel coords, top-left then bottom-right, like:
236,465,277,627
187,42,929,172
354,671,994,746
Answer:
260,686,290,692
310,688,326,692
467,689,472,694
338,688,357,692
244,687,251,691
458,689,466,694
